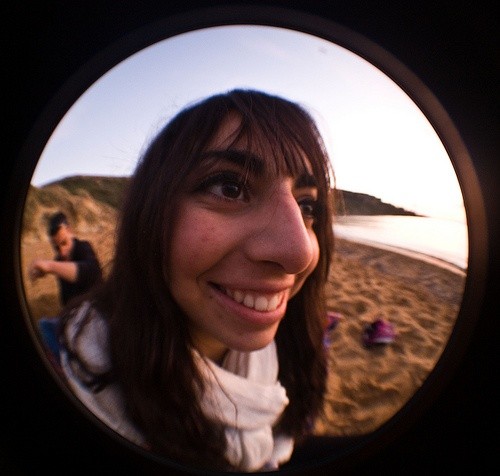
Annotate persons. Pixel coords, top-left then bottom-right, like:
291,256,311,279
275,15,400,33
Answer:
58,90,335,469
22,209,102,356
